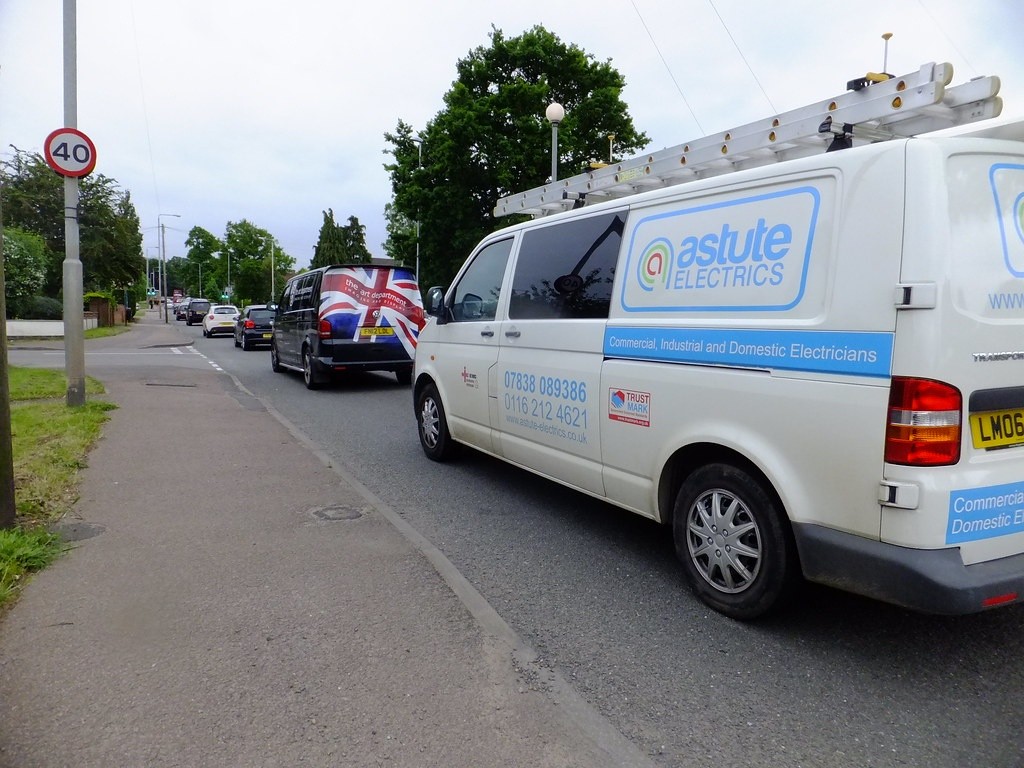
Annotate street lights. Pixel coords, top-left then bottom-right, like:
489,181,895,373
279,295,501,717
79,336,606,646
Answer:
192,262,201,298
409,136,423,282
882,32,893,73
258,237,275,302
158,213,181,319
607,135,615,162
146,247,158,286
218,252,230,306
546,103,564,183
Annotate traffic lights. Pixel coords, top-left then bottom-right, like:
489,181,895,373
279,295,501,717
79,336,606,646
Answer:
222,292,224,299
152,288,154,295
153,272,159,290
225,292,228,298
147,289,150,295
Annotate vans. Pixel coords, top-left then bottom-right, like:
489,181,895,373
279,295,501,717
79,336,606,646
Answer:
412,62,1024,618
266,264,424,390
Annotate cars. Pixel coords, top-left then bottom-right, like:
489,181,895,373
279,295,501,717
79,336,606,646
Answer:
201,306,241,338
164,297,183,314
175,304,189,321
184,301,211,326
233,305,279,351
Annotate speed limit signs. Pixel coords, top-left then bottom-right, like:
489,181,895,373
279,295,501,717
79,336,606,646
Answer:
45,128,97,177
